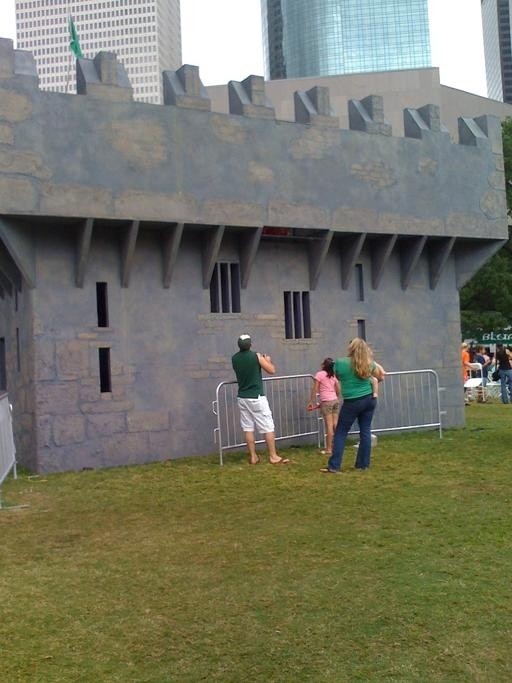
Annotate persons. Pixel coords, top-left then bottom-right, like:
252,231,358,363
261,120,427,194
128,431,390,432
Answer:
306,358,340,454
461,342,512,406
232,335,289,464
371,363,385,398
320,338,384,473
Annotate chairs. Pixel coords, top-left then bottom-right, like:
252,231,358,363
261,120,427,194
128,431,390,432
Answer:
464,363,485,403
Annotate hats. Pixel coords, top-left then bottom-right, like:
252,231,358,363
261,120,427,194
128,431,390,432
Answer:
238,333,251,349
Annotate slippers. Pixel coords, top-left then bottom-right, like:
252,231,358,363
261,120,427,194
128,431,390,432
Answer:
320,449,331,454
249,455,262,465
270,457,289,464
321,468,337,472
